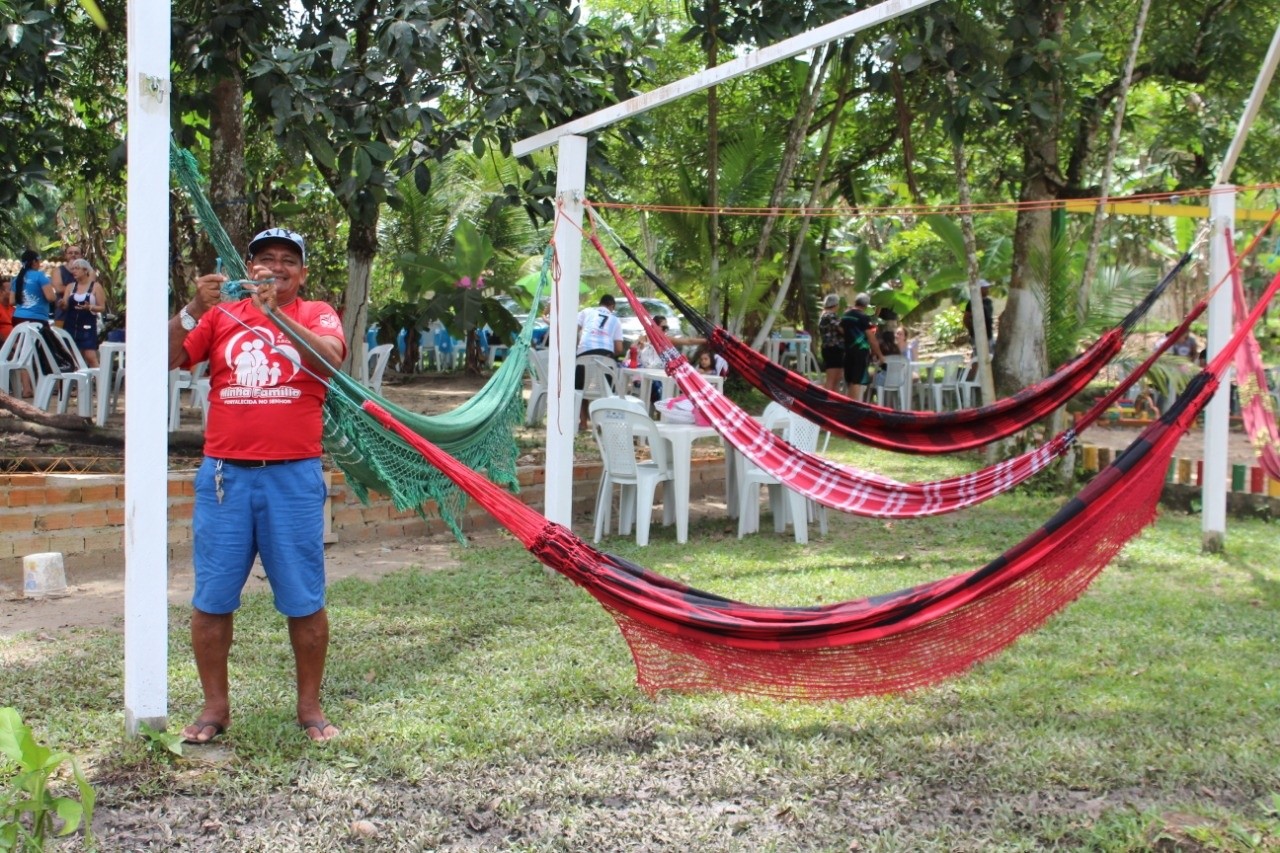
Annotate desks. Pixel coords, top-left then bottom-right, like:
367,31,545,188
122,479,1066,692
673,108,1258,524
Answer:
635,413,766,542
618,364,724,422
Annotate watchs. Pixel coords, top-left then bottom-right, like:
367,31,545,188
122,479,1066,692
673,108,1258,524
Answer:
181,305,199,331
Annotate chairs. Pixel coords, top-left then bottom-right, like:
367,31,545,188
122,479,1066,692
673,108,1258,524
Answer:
523,335,1280,546
364,320,548,397
0,322,211,434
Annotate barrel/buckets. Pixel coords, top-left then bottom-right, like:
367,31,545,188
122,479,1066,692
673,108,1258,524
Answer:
22,553,72,599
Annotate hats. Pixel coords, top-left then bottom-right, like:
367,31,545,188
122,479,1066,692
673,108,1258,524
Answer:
879,308,897,321
249,228,305,265
69,259,93,275
979,280,993,287
1165,325,1181,337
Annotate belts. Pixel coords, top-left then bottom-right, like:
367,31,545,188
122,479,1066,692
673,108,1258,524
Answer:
208,456,307,469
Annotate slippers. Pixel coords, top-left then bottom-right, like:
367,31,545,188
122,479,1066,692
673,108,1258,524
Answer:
296,719,331,749
185,718,226,744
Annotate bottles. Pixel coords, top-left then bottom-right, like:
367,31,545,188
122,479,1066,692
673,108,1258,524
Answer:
629,346,637,368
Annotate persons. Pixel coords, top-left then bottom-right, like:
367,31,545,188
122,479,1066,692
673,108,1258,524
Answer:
169,229,347,745
1,244,106,398
541,279,994,405
1158,326,1206,367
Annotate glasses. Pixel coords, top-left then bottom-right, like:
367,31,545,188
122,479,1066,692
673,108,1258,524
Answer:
660,325,669,332
71,266,83,271
